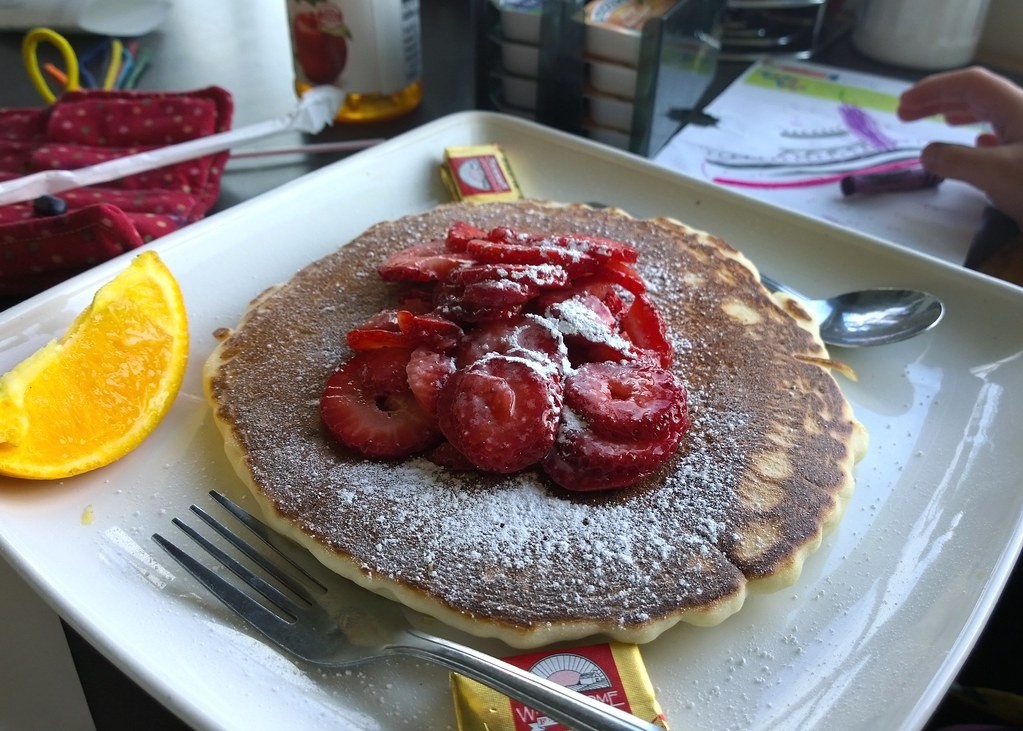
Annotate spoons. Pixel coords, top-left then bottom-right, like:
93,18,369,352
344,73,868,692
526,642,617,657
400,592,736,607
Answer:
758,273,945,349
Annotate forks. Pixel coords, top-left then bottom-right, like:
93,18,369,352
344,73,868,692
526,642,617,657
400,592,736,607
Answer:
150,488,664,730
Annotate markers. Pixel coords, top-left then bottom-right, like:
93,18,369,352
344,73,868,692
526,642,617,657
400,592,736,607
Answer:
839,168,945,196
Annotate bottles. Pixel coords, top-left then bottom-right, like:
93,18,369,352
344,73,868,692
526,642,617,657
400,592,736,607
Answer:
286,0,424,124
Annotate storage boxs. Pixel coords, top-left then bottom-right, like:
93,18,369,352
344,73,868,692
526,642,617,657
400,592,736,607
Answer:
475,0,722,159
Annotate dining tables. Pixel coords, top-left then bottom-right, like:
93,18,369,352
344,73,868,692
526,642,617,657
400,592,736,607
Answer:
61,551,1023,731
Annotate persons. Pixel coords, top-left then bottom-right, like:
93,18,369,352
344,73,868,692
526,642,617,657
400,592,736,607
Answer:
899,64,1023,227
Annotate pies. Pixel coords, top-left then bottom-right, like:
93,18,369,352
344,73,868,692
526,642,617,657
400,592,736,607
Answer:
204,197,867,651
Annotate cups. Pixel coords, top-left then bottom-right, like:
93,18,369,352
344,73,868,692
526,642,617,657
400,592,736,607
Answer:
847,1,991,70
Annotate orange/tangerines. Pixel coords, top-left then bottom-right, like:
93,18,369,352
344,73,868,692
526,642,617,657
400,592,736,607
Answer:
1,250,190,482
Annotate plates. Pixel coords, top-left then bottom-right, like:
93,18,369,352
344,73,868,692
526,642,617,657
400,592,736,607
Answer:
0,110,1022,731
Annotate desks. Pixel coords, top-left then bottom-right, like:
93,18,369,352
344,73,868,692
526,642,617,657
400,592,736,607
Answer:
664,55,1023,280
